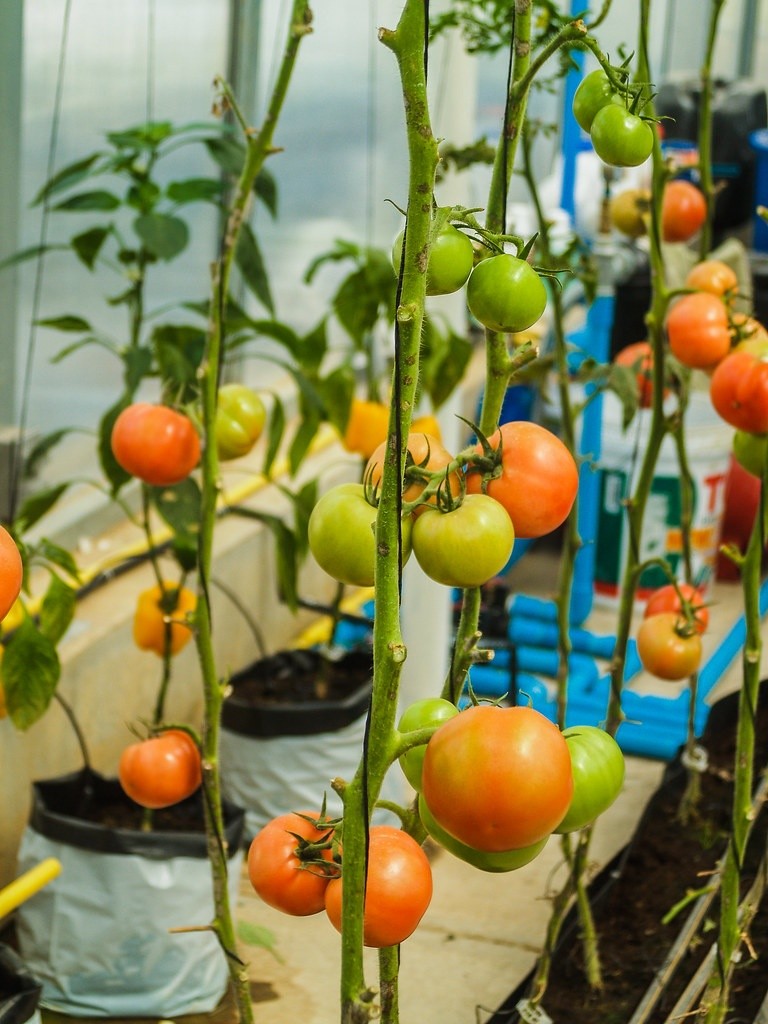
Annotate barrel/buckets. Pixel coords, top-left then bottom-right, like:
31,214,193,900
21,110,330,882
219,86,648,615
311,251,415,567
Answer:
540,369,736,616
750,130,768,252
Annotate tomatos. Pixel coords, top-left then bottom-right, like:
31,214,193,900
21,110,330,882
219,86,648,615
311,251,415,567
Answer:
109,66,768,948
0,523,23,624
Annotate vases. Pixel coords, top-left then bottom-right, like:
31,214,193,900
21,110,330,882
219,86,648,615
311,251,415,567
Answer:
14,767,246,1024
220,640,372,841
